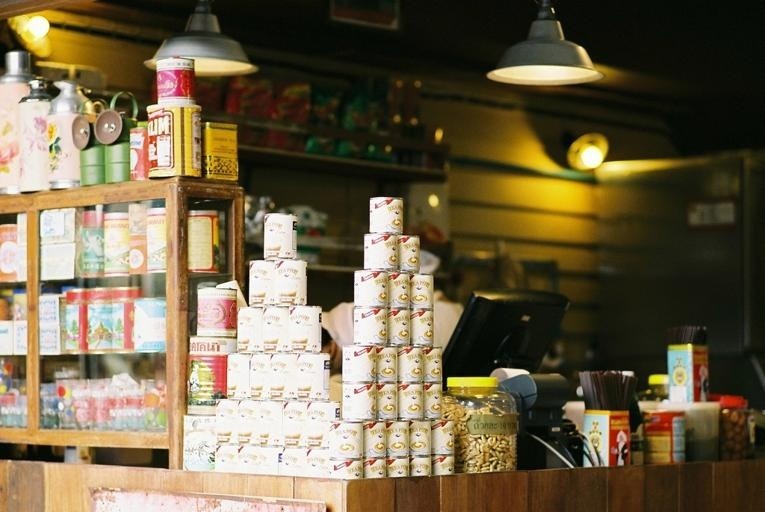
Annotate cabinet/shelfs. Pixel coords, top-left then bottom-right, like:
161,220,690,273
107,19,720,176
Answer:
0,174,244,470
44,62,468,278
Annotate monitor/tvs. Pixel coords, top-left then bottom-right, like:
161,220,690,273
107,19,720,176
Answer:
443,288,570,392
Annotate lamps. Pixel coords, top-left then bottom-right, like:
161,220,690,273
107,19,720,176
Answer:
559,124,610,171
486,0,604,86
142,0,259,77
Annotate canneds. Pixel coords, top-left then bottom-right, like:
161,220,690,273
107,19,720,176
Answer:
354,307,434,346
248,260,307,308
364,235,420,269
354,270,435,307
227,353,331,403
263,213,298,261
642,409,687,466
63,286,167,355
187,336,237,415
102,212,130,278
342,346,443,382
342,383,443,419
328,419,455,454
197,288,237,338
184,416,216,472
145,207,168,274
328,455,455,479
215,399,340,477
237,306,323,354
370,197,404,235
129,58,205,181
186,209,220,274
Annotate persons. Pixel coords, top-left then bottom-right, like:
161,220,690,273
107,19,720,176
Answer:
419,248,465,355
320,301,354,374
